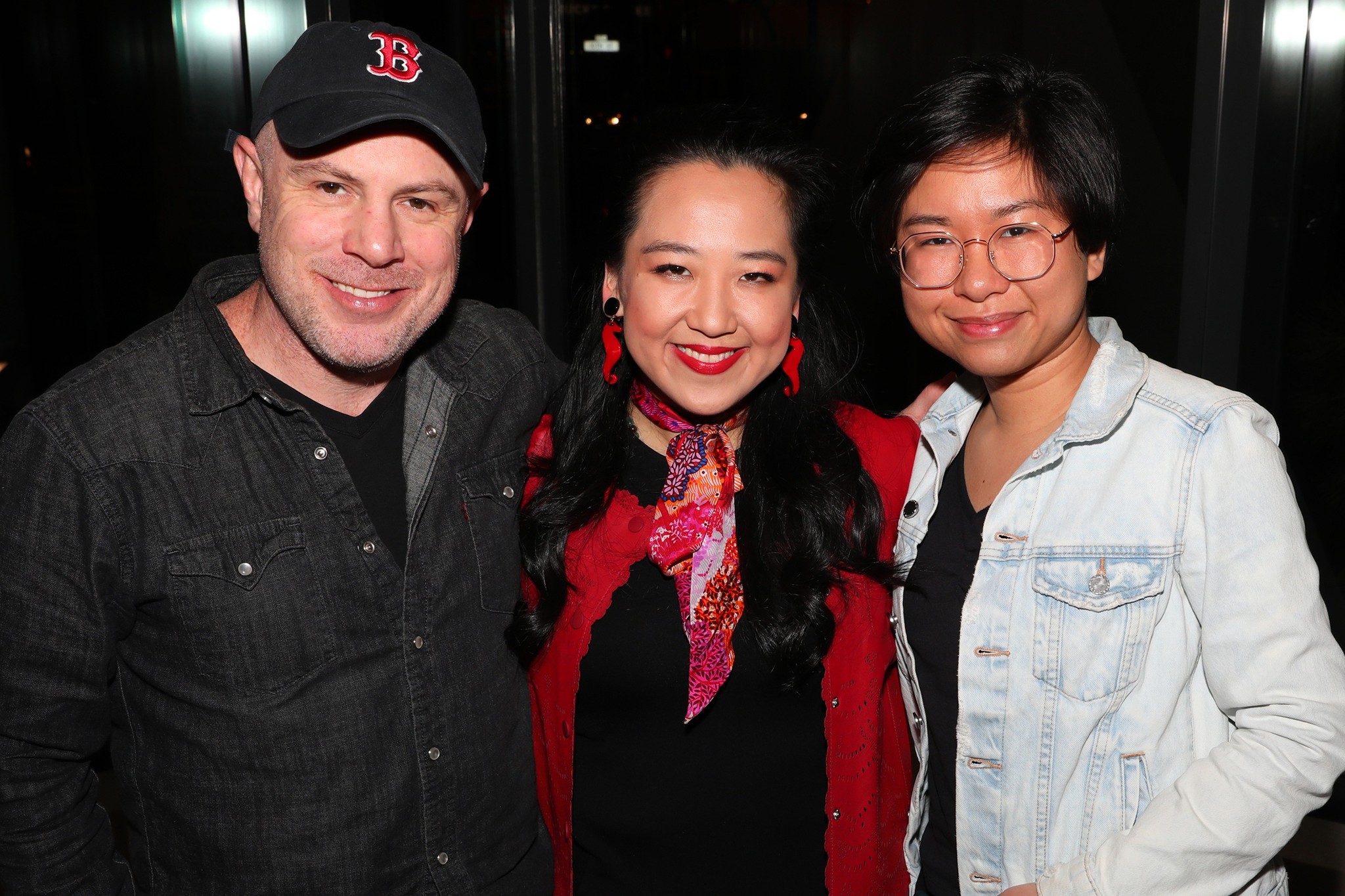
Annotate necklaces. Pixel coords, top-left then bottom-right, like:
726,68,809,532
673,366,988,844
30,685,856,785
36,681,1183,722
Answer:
626,415,640,440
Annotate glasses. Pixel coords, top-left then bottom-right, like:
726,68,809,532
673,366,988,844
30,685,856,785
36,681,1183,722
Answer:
889,221,1075,288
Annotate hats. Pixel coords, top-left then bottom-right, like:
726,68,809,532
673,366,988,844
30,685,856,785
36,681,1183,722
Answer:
224,22,488,191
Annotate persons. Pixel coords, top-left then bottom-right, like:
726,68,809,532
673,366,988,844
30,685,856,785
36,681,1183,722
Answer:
864,58,1344,895
0,14,958,896
505,124,918,896
